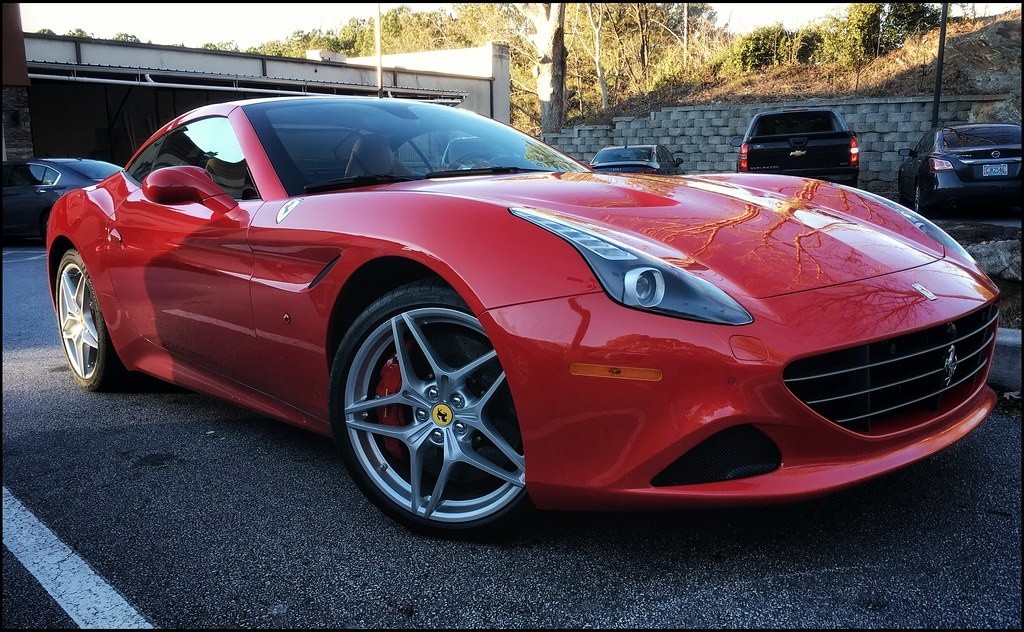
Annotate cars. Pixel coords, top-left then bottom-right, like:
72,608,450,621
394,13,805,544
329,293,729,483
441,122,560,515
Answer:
590,145,687,174
2,157,124,240
897,121,1021,218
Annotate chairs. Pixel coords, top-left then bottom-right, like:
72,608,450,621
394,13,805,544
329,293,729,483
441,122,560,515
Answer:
344,134,415,182
208,159,247,202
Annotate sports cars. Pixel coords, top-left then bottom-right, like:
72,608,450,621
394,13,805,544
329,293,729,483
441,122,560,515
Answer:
48,96,1001,544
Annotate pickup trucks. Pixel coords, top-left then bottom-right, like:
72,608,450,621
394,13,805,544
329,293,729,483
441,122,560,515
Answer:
729,108,860,189
